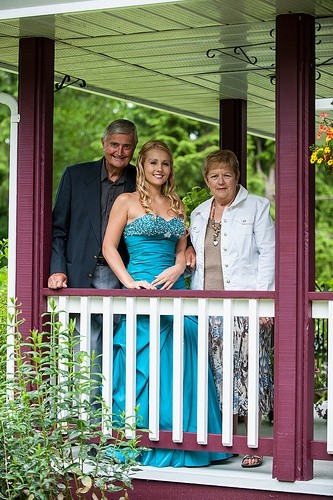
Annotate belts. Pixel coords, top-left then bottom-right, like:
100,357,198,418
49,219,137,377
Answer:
96,257,108,266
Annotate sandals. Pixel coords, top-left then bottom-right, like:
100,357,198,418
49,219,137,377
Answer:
242,452,264,468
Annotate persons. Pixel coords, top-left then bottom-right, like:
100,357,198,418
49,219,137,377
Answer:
188,149,277,467
47,120,196,439
84,139,234,469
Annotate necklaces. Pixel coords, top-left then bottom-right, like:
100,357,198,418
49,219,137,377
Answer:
211,197,235,246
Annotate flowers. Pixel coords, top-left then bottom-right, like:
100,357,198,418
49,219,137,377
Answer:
309,112,333,175
